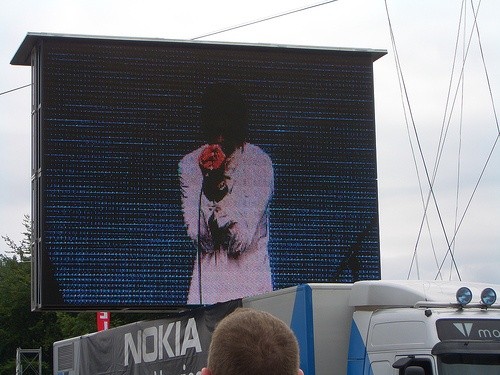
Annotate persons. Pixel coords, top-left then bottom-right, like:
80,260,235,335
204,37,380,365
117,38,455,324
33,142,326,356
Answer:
197,309,306,375
178,83,275,306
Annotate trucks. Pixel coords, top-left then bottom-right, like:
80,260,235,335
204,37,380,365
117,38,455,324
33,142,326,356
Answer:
79,281,500,375
